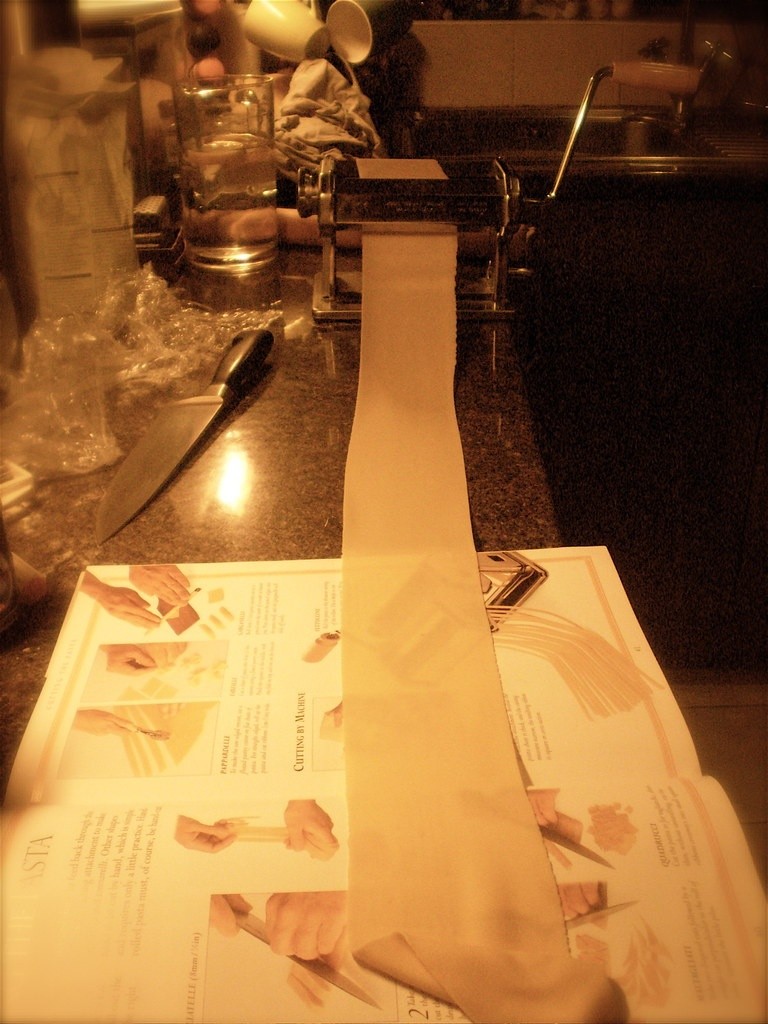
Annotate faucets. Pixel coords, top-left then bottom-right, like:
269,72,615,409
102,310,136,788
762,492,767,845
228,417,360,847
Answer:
639,0,731,118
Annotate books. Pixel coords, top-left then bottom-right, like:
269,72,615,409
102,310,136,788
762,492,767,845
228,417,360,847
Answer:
0,545,768,1024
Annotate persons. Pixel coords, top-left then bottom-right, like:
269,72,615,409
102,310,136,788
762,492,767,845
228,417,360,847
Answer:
81,564,627,1024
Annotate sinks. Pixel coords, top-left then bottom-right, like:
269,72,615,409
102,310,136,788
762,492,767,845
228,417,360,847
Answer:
398,105,724,164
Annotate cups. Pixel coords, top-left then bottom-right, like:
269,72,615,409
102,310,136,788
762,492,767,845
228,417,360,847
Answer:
325,0,413,63
172,73,280,275
243,0,329,61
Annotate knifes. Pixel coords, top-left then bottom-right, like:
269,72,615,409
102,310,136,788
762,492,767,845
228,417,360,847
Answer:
95,331,275,544
